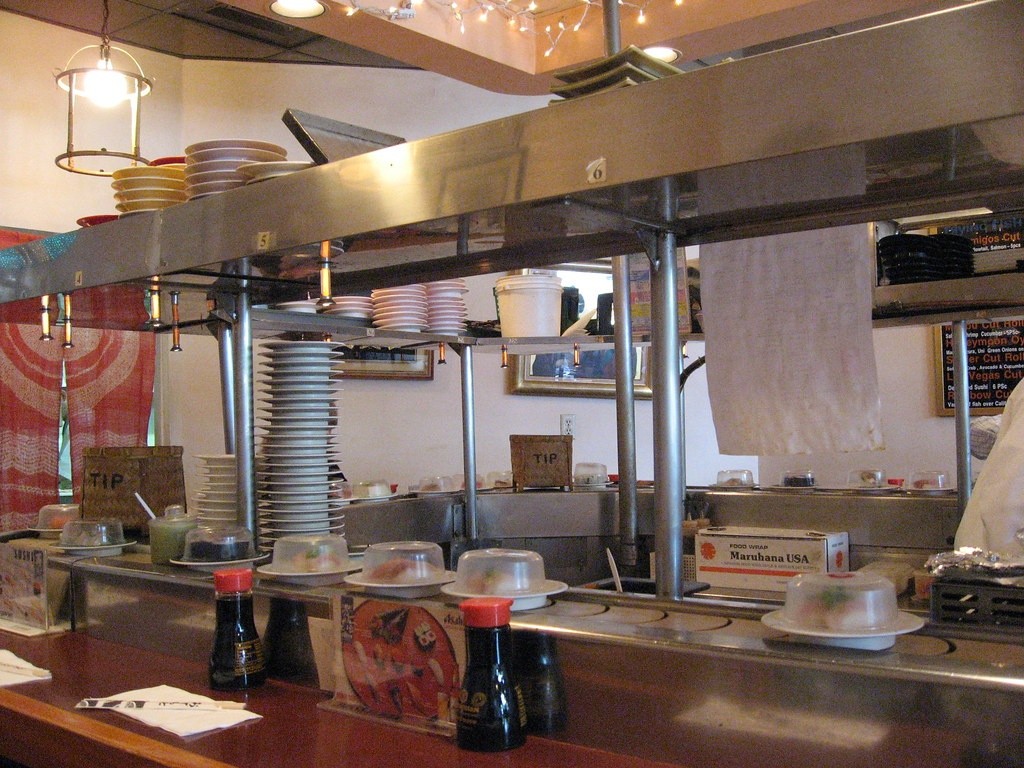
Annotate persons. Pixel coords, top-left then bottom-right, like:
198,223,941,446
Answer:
953,377,1024,560
687,266,703,333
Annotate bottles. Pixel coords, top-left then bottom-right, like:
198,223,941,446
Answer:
262,594,318,688
456,598,529,753
512,630,567,733
209,567,267,691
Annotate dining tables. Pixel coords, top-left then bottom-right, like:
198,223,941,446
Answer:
0,629,680,768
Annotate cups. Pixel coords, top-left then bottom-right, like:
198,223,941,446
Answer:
148,515,198,567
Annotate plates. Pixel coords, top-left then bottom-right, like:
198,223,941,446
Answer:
278,278,470,336
843,484,899,495
113,139,316,218
49,540,138,557
905,487,953,496
167,342,367,545
358,494,399,501
547,45,685,104
876,233,976,285
260,558,363,587
442,580,568,612
27,527,63,539
167,551,269,573
761,609,925,651
410,486,512,496
771,484,818,492
573,482,614,489
710,484,760,491
343,570,456,598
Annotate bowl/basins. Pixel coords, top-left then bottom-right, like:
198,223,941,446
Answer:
608,474,619,483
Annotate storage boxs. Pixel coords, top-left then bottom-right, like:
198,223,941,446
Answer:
694,526,850,593
930,574,1024,628
78,444,188,535
510,435,573,492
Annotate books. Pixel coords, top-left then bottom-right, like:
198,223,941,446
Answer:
610,246,703,335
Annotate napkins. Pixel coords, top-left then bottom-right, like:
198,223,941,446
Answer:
102,683,264,737
0,648,53,687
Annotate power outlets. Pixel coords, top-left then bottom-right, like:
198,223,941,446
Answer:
560,414,577,440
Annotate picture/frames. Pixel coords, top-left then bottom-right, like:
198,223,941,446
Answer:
293,330,436,381
506,257,654,400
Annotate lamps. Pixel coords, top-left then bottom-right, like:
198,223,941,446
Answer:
50,0,153,177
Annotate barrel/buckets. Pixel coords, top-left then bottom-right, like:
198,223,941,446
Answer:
496,275,564,338
